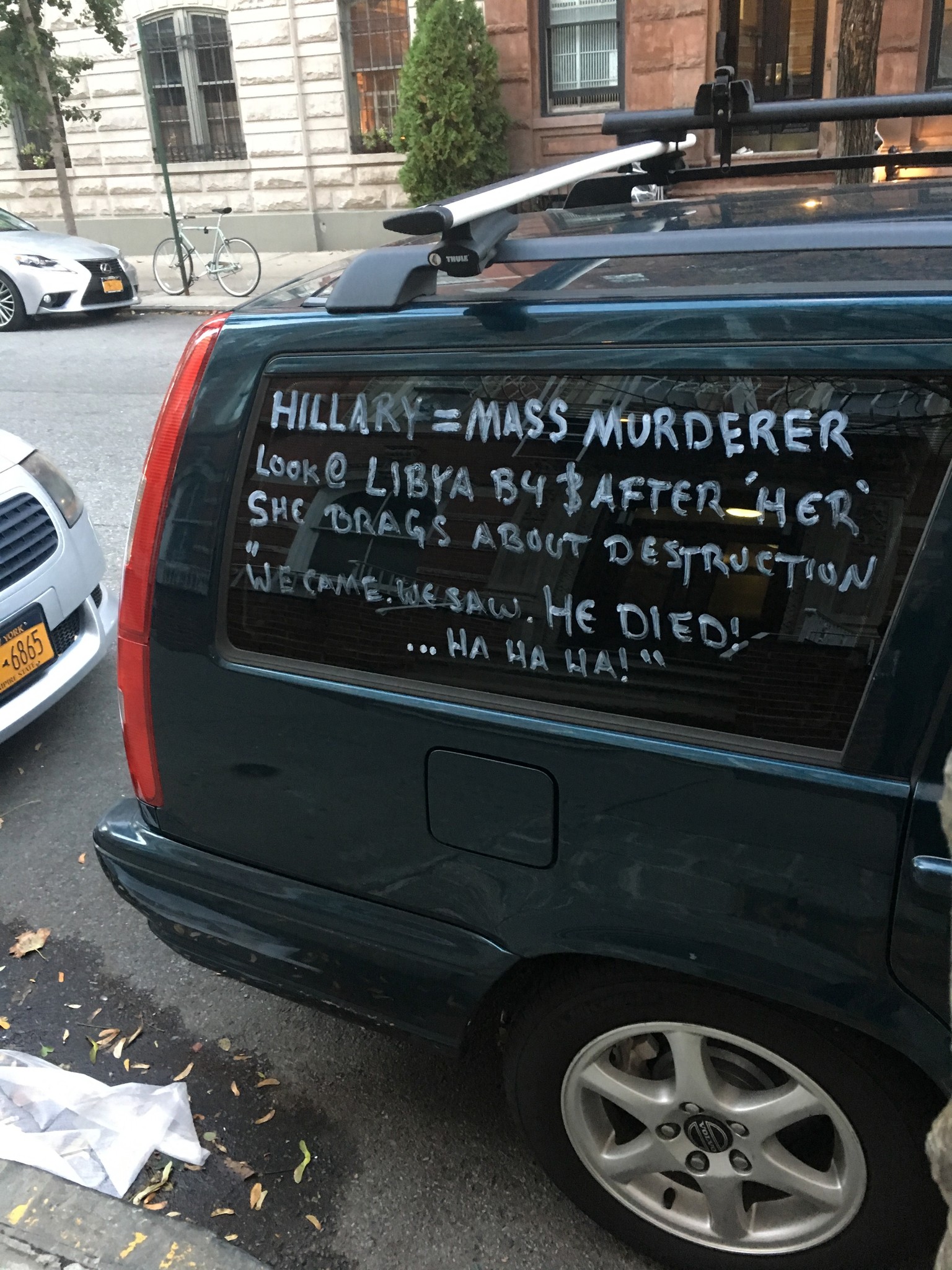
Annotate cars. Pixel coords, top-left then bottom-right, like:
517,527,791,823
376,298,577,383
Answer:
0,427,119,746
0,209,143,333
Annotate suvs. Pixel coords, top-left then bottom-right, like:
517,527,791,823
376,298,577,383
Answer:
89,61,952,1270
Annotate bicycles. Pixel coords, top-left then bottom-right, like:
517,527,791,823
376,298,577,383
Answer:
152,207,262,298
